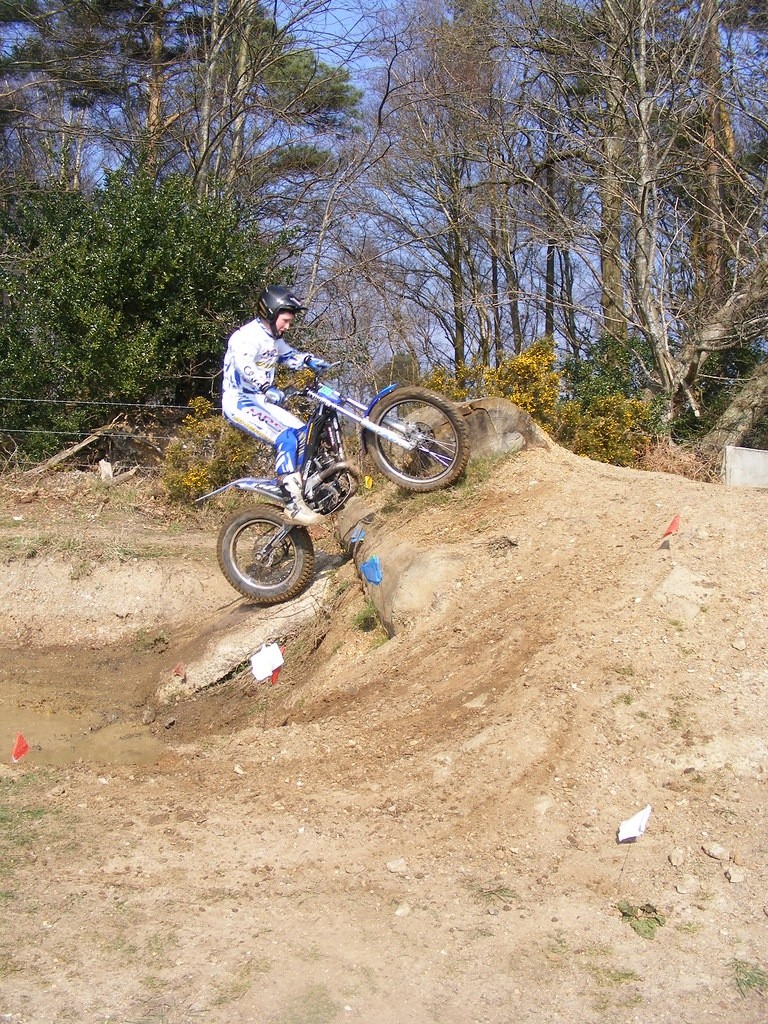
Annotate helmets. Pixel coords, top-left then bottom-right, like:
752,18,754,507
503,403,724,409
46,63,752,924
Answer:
259,285,307,324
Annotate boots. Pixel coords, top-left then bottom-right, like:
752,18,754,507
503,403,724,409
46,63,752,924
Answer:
278,472,326,527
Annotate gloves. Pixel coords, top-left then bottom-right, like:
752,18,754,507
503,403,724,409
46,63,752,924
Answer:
307,357,331,373
264,386,286,405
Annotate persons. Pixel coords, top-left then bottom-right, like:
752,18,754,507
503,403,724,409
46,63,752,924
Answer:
221,285,330,525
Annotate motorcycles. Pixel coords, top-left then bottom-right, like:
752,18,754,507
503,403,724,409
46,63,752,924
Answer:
195,355,472,603
